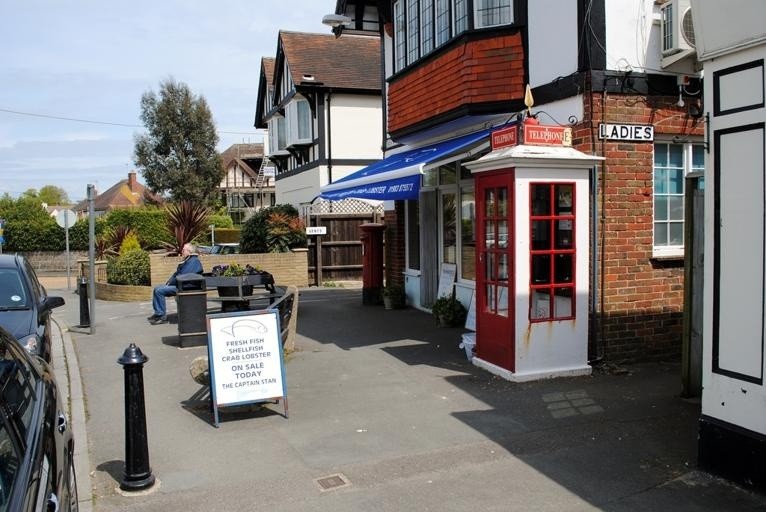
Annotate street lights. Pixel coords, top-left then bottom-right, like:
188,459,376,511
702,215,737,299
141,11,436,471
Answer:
206,223,214,246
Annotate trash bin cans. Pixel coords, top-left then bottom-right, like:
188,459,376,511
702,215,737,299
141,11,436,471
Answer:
175,273,208,348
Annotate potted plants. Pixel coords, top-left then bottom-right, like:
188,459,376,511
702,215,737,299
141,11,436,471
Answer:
431,294,468,328
382,283,407,310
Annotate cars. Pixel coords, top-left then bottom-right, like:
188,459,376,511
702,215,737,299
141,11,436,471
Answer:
0,249,66,364
486,231,508,249
0,356,34,430
195,244,211,254
0,323,81,512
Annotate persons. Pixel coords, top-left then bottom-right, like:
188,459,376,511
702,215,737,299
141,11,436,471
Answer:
147,242,204,325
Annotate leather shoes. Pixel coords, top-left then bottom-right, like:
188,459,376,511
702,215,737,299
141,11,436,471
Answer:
151,315,168,325
148,314,158,320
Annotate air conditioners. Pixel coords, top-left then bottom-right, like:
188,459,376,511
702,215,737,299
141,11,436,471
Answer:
660,0,696,56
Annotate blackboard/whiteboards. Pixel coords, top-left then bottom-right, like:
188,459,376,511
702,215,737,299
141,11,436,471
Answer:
205,308,287,408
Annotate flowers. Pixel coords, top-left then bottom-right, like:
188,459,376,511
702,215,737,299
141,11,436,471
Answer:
211,262,263,277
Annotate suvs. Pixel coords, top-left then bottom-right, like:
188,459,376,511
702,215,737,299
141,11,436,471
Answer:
208,240,240,254
